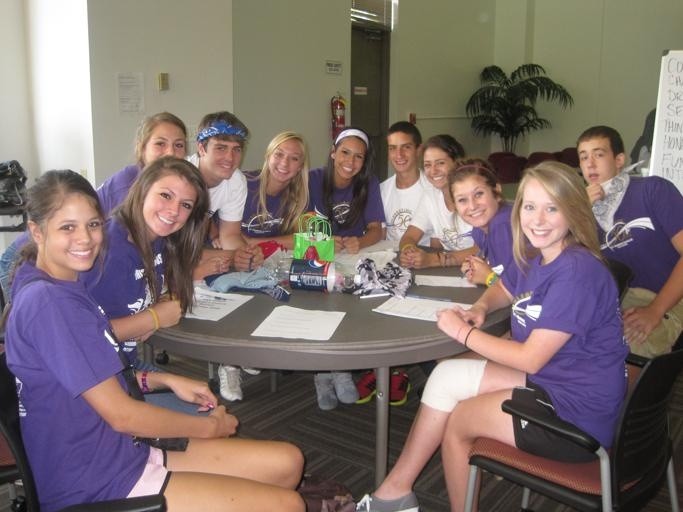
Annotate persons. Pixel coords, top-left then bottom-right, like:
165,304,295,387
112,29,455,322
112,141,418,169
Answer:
354,159,632,512
573,124,683,362
77,155,210,375
1,166,309,511
1,110,233,359
433,157,534,363
184,110,266,404
306,125,385,411
395,132,481,272
202,132,311,253
352,121,428,409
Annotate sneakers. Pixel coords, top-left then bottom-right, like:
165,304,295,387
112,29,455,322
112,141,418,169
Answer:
218,365,261,402
356,493,419,512
314,371,411,411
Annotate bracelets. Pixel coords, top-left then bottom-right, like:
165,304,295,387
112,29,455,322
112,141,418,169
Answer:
464,326,478,349
400,243,416,252
439,251,448,267
147,305,162,330
141,369,149,391
486,271,498,286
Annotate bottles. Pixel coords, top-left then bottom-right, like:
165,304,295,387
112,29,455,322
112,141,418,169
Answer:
274,256,362,291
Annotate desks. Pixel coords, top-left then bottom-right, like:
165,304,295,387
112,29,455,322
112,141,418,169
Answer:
498,183,525,204
140,247,511,496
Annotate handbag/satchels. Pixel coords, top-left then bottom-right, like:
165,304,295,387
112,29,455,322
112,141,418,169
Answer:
618,286,683,359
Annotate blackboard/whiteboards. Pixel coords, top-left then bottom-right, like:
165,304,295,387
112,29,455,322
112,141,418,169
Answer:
649,49,683,192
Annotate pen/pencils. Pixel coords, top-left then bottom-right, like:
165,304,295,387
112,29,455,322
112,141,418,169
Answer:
200,294,225,301
360,294,390,299
461,250,481,279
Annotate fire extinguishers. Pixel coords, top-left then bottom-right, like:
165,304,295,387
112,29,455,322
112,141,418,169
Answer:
331,92,347,139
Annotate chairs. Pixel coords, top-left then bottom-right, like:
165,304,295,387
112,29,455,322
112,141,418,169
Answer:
488,109,656,186
463,348,683,512
606,258,633,307
